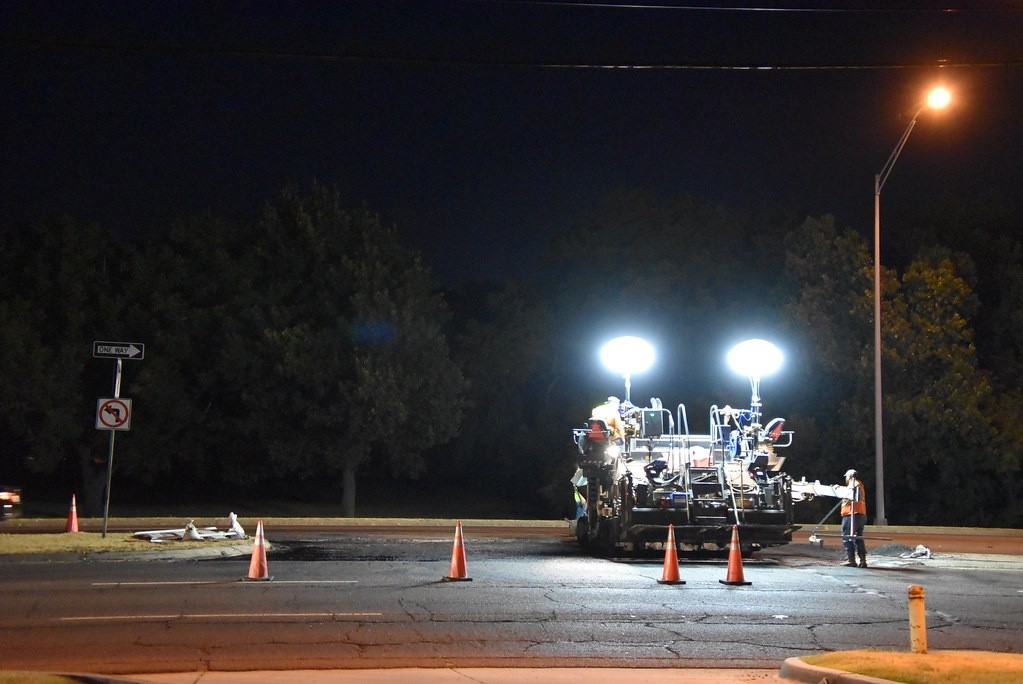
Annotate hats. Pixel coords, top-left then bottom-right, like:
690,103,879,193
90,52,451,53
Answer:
844,469,859,478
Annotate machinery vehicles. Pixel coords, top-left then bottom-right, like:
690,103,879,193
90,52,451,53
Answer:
571,397,892,558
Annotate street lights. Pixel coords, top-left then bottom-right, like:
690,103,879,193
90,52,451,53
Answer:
874,88,951,525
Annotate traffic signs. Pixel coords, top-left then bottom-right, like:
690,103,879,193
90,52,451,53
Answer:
93,341,144,360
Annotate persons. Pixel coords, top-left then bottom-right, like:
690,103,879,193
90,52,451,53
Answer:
834,469,868,567
590,396,625,452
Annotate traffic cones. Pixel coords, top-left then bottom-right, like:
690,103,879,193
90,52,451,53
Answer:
656,524,686,585
443,520,474,582
719,524,753,585
242,519,274,582
66,494,79,533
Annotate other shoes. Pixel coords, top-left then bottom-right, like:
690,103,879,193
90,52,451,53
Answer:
858,562,867,568
839,560,857,567
